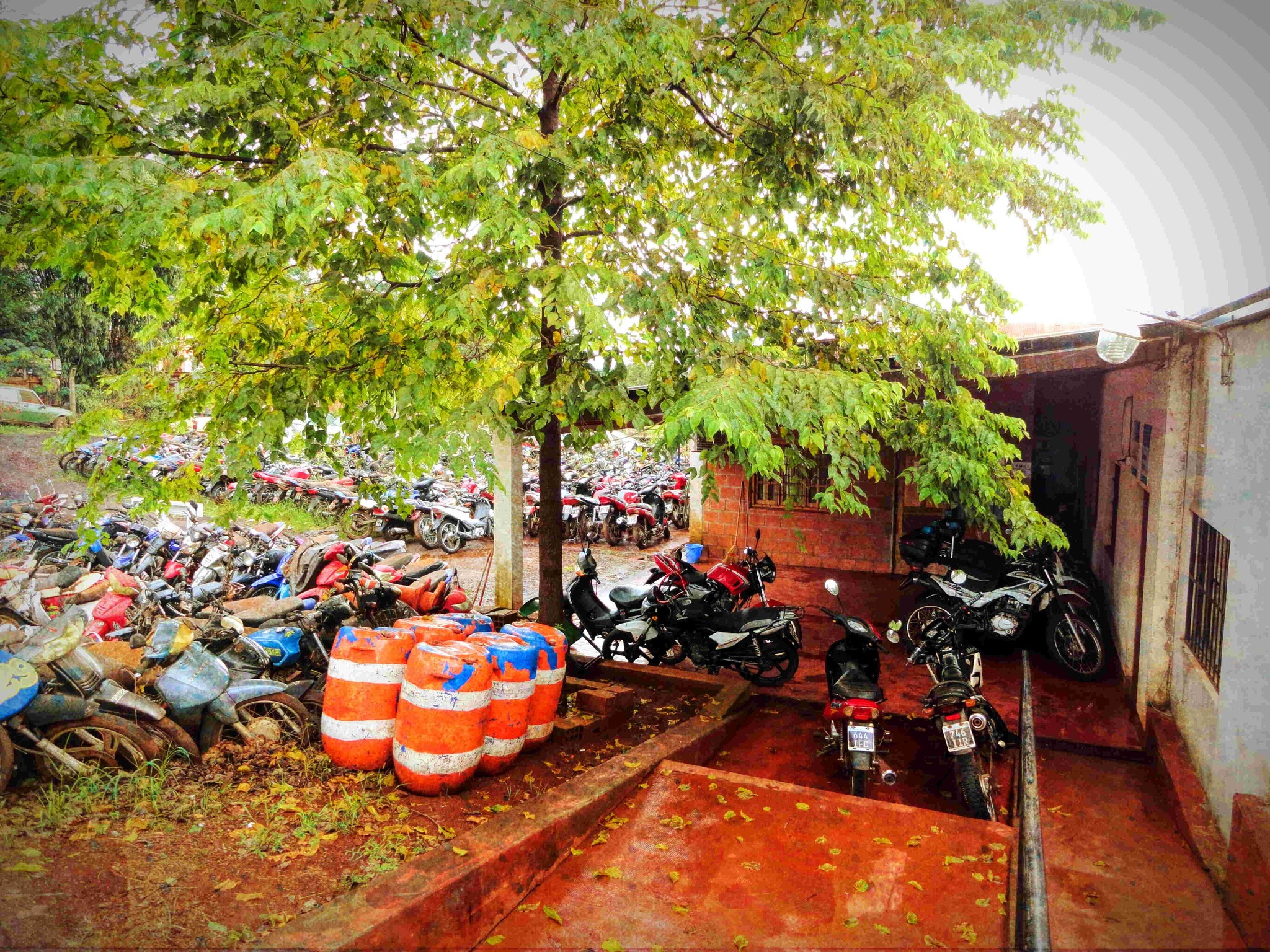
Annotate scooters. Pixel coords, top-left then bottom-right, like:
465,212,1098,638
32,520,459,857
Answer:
0,419,688,804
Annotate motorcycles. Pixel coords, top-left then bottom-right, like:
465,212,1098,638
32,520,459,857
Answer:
903,566,1021,823
818,578,897,798
644,529,802,652
899,515,1113,683
602,548,806,688
561,536,687,664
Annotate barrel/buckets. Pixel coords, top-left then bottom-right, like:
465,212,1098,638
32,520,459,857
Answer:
321,626,417,771
498,621,568,753
428,613,496,636
392,640,493,796
682,544,704,563
393,616,465,646
465,632,538,777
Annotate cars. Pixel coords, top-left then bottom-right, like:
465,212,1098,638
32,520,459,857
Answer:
0,383,75,429
